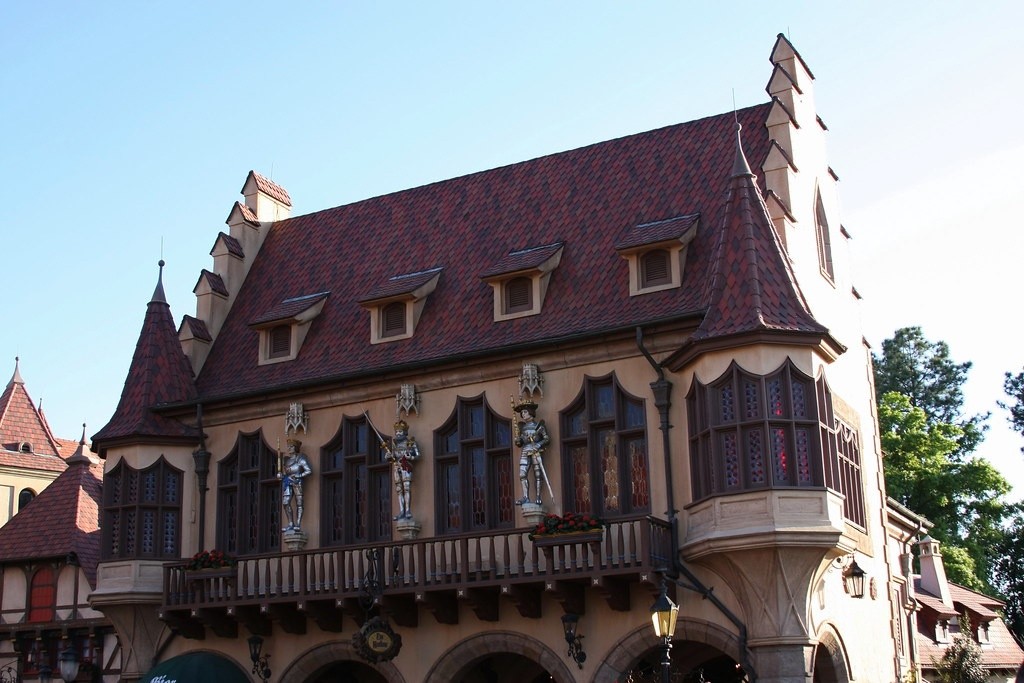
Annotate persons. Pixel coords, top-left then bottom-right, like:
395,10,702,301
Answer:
277,439,312,531
514,399,550,505
384,420,420,521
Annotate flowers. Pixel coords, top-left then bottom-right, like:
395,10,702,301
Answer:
180,548,238,569
523,511,612,543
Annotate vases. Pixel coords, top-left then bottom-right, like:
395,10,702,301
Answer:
185,564,238,580
531,527,604,545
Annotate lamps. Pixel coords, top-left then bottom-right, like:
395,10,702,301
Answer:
246,635,271,683
834,551,866,597
561,613,585,669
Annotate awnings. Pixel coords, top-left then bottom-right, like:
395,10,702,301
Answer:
138,651,251,683
